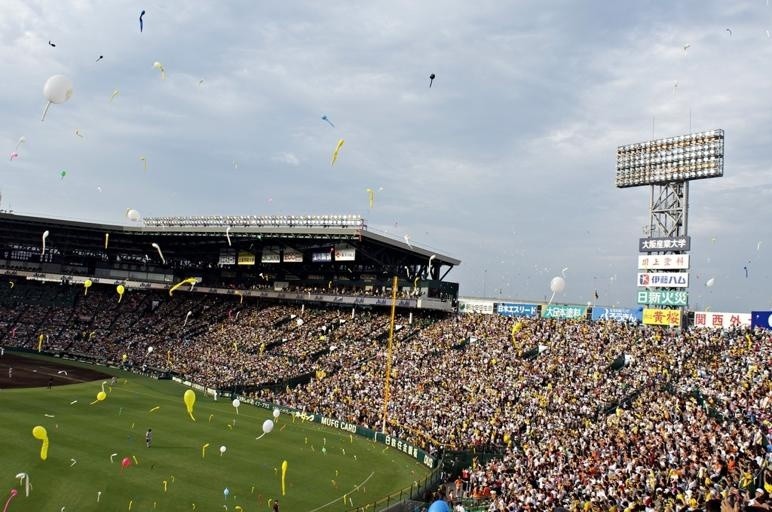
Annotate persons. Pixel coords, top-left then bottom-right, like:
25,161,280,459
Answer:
1,252,772,512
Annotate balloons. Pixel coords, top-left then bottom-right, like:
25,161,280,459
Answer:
2,8,440,255
